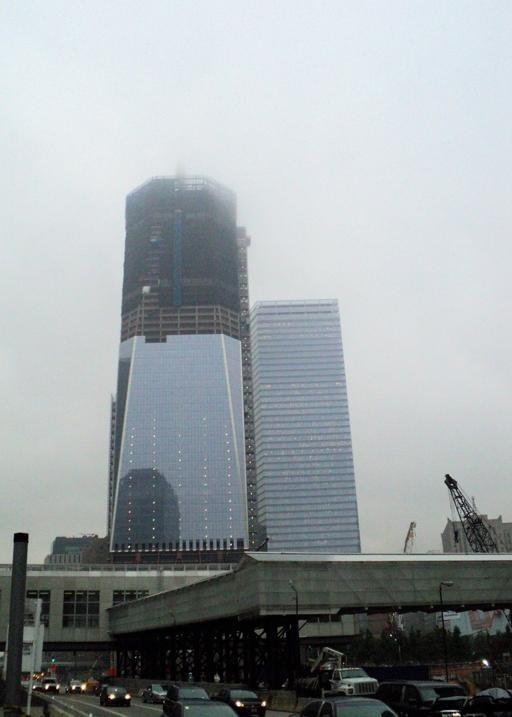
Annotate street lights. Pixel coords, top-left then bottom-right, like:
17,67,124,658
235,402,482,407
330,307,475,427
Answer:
438,580,454,679
287,578,299,621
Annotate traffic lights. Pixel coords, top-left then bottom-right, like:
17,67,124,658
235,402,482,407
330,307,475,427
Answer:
50,657,56,670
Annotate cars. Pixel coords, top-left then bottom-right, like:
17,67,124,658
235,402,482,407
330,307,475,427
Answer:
22,674,132,708
